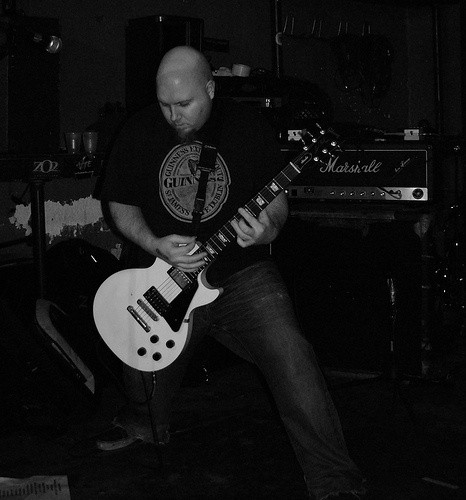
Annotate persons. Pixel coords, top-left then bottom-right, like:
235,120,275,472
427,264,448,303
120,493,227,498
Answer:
90,46,374,500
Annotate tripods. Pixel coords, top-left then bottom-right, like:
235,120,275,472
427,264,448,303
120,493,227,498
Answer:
384,319,424,436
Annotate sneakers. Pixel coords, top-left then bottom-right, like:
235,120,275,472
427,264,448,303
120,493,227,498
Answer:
97,426,138,451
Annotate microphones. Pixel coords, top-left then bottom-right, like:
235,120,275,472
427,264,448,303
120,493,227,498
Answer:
383,272,403,318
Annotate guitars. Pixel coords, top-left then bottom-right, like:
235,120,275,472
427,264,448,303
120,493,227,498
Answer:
94,121,346,373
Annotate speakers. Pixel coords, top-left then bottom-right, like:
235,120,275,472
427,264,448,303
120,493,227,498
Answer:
0,13,61,154
125,13,204,119
269,202,431,384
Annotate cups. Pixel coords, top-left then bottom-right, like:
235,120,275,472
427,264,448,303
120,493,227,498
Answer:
65,132,80,153
83,132,97,154
